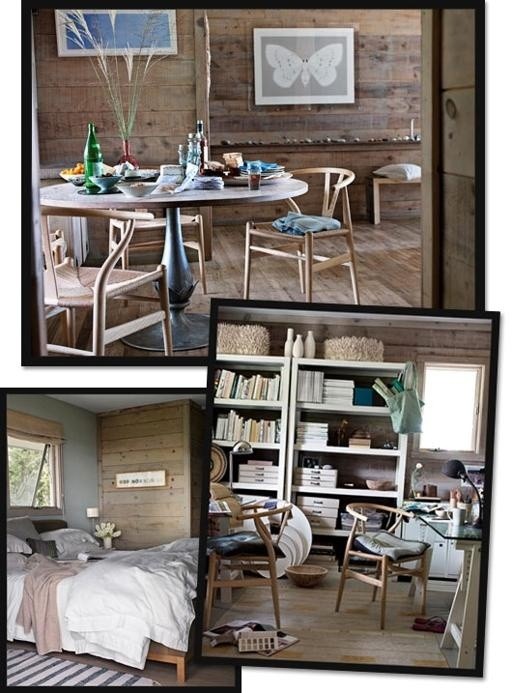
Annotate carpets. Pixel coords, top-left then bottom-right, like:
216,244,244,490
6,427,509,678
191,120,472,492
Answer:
4,643,161,689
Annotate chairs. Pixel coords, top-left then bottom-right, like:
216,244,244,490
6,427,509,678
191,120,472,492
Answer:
100,208,209,307
331,500,432,628
206,481,299,653
243,166,362,306
40,214,173,357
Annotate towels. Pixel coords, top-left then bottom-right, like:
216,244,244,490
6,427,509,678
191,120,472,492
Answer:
271,210,341,235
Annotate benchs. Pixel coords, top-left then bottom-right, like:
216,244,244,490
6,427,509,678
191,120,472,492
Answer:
362,174,422,223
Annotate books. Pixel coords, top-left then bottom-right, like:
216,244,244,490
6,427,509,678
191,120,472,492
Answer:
54,551,108,564
202,617,301,658
208,364,355,446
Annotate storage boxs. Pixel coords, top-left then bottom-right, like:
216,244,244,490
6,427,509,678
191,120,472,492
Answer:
352,387,373,405
348,437,372,449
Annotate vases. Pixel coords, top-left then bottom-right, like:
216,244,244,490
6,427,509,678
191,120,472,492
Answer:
111,135,140,169
102,537,112,548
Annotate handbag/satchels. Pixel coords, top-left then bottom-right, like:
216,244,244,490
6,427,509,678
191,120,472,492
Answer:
386,361,425,434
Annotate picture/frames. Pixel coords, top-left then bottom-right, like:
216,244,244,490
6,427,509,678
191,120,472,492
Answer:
50,8,180,60
249,24,357,107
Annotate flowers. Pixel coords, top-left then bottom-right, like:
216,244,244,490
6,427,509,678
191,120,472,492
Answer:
92,521,122,539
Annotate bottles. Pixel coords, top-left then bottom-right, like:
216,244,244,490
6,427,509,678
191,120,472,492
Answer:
83,120,104,187
178,119,208,173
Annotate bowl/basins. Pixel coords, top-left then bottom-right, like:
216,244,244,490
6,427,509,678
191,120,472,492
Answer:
116,180,160,196
58,170,86,185
365,480,393,490
88,173,123,189
434,508,452,518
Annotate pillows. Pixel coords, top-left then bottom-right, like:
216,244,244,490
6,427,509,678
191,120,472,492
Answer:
372,162,422,180
7,516,100,563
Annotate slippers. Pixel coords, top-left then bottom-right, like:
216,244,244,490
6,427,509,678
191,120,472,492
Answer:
412,615,446,633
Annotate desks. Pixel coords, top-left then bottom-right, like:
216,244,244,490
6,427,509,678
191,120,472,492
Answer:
206,511,247,602
418,506,484,672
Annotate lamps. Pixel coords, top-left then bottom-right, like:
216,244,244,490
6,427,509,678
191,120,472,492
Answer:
436,458,487,541
85,506,102,547
228,438,255,495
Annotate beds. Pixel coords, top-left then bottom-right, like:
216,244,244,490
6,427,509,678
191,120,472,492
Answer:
6,525,205,686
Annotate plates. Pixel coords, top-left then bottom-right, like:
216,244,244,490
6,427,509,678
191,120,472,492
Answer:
116,167,158,181
433,517,450,520
226,165,295,186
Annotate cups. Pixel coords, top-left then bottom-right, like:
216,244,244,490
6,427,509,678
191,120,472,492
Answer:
247,167,262,192
446,507,467,527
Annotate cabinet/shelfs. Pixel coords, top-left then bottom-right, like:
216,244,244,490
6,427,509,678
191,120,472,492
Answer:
214,354,289,555
288,357,415,564
399,509,477,581
38,177,89,268
